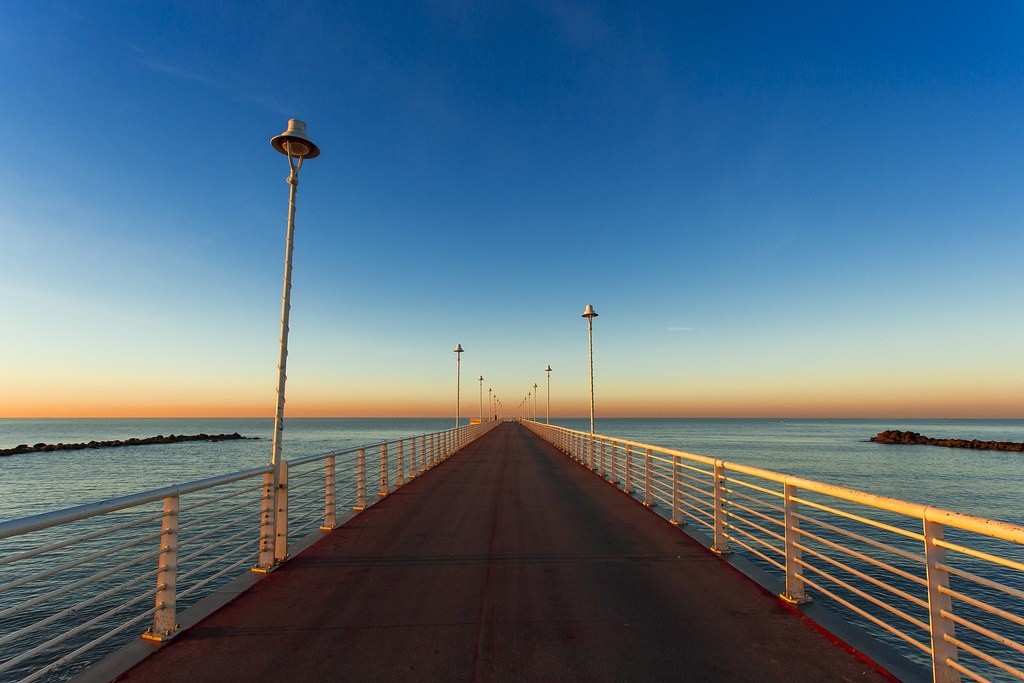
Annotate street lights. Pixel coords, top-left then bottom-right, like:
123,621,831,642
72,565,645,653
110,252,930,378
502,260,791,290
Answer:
524,396,527,419
494,395,496,419
488,388,492,421
544,365,552,424
528,391,531,420
269,119,320,465
478,375,484,423
454,344,464,428
533,383,537,422
581,304,599,434
